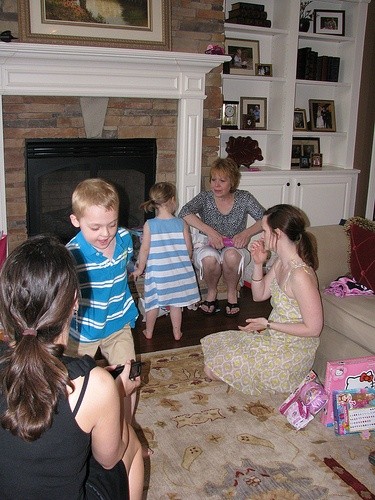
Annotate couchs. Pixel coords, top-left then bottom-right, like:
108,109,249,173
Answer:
298,224,375,386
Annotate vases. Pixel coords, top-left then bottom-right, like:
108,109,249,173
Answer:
299,18,310,32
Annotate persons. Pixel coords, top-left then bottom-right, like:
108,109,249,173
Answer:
176,156,268,316
199,204,323,396
63,178,139,426
249,106,260,123
302,159,307,166
315,104,332,129
258,67,270,75
0,236,144,500
127,182,201,340
234,48,242,67
324,18,336,30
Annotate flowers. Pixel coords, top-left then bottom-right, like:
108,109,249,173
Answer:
300,1,313,19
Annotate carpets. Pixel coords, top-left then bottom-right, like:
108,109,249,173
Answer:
96,344,375,500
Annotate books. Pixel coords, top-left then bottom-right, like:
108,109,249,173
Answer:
296,46,341,82
225,2,272,28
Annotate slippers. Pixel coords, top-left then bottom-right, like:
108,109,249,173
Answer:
201,298,219,314
225,302,239,317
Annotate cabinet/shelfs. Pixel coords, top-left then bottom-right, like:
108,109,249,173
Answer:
127,262,241,317
221,0,371,287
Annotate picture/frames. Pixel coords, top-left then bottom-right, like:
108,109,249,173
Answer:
294,110,307,131
224,38,272,77
222,96,267,130
308,100,336,132
19,0,172,51
313,9,345,36
292,137,322,169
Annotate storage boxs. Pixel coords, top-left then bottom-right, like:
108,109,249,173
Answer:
320,355,375,427
332,388,375,435
226,2,271,28
279,370,330,431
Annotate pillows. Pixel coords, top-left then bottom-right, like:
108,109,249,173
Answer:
345,216,375,294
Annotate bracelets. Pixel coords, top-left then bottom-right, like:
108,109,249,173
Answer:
251,274,263,282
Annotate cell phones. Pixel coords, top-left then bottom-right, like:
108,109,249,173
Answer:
110,361,142,380
